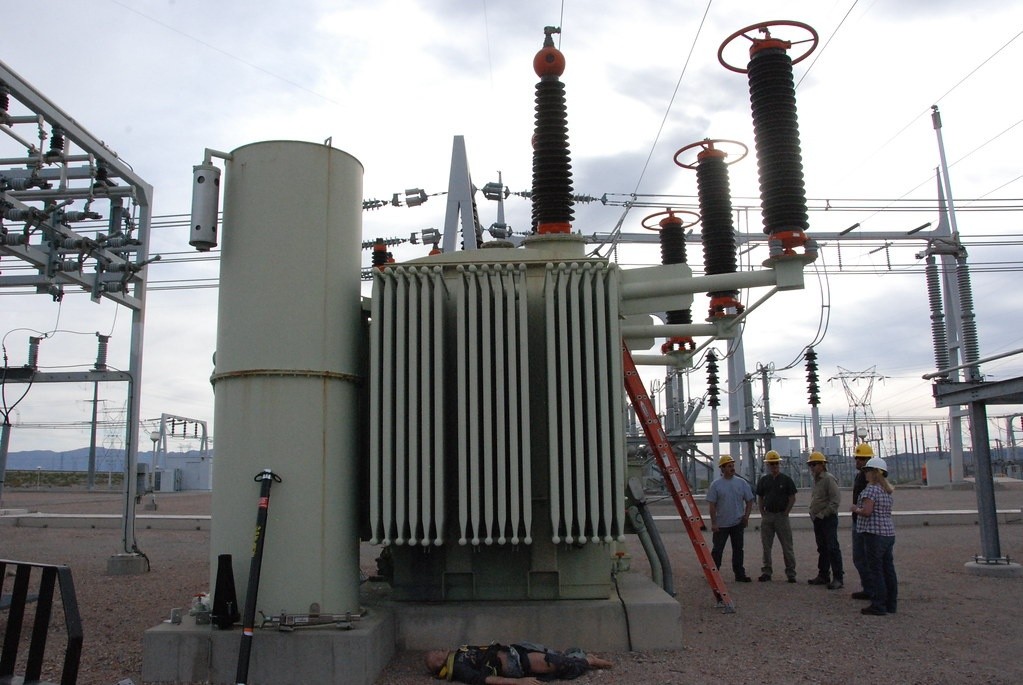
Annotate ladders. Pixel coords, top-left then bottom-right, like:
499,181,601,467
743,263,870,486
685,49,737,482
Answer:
622,337,735,614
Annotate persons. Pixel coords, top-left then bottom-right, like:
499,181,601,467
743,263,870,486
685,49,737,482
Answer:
851,444,876,600
756,450,798,582
807,451,845,590
850,457,898,617
426,640,614,685
704,455,753,583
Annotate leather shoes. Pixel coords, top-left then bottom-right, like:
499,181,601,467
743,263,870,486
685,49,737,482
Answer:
851,590,873,600
759,575,769,582
788,577,797,583
860,606,883,615
827,581,844,589
808,576,830,584
735,574,751,582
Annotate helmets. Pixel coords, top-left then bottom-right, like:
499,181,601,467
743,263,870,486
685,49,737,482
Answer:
718,455,734,466
854,444,876,457
861,456,889,477
807,452,828,464
763,450,783,463
